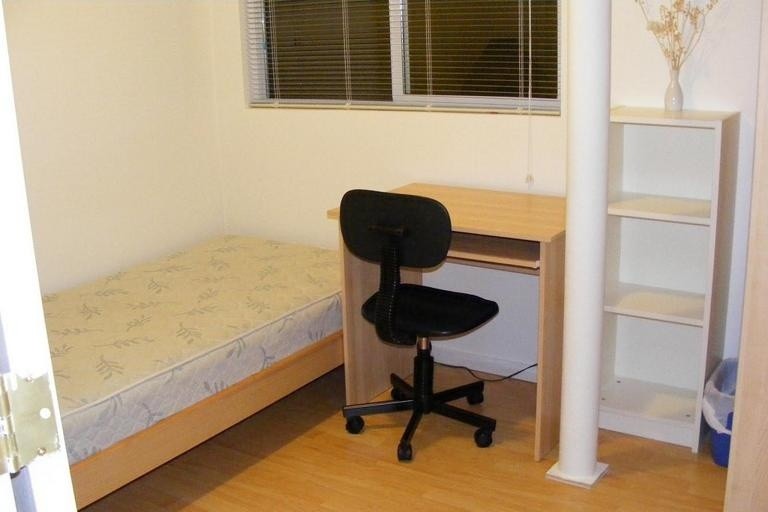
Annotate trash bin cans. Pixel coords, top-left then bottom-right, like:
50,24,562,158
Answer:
703,358,739,468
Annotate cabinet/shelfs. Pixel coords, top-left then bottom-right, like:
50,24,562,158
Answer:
598,106,740,454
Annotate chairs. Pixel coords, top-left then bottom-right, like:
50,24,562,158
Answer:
340,190,498,462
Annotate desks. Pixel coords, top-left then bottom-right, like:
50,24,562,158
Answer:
327,182,565,463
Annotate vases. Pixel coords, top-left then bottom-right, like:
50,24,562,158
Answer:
665,69,683,112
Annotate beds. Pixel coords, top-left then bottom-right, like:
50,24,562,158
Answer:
43,235,344,512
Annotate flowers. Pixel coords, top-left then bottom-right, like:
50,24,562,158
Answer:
636,0,718,69
